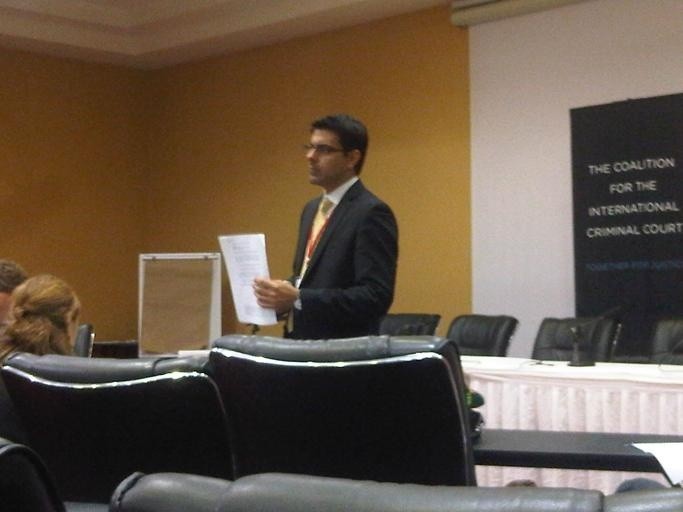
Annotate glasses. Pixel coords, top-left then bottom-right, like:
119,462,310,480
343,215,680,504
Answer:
305,143,348,155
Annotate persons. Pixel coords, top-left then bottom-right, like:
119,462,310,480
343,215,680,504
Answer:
252,113,398,339
0,262,27,317
0,273,82,364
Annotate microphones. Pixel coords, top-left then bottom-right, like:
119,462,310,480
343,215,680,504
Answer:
567,305,625,367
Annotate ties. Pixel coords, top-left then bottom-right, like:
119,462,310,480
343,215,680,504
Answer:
287,199,333,334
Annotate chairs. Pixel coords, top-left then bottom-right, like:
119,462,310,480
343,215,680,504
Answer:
0,311,680,512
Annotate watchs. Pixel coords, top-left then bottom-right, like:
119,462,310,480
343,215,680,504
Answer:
294,289,302,312
